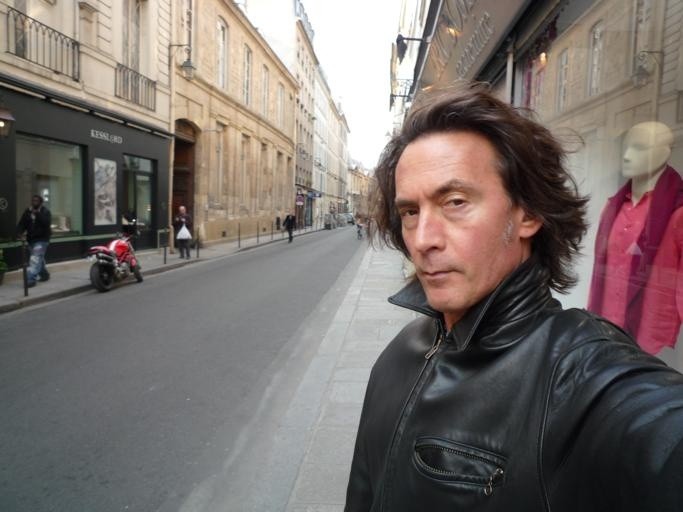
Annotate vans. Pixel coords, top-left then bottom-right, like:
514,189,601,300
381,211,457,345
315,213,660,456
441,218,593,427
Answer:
344,213,355,225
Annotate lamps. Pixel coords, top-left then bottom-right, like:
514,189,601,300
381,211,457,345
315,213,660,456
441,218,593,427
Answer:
170,43,197,81
396,35,427,63
0,109,15,137
390,94,411,109
631,50,664,89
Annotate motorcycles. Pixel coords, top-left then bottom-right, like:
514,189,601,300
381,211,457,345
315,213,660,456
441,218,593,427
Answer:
81,219,142,292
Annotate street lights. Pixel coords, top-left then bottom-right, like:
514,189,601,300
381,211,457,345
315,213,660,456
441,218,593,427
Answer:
168,42,195,255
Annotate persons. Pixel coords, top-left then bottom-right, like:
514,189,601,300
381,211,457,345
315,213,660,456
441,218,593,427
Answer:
341,77,682,511
356,217,363,237
172,205,191,259
281,209,296,243
14,193,51,288
580,118,681,357
